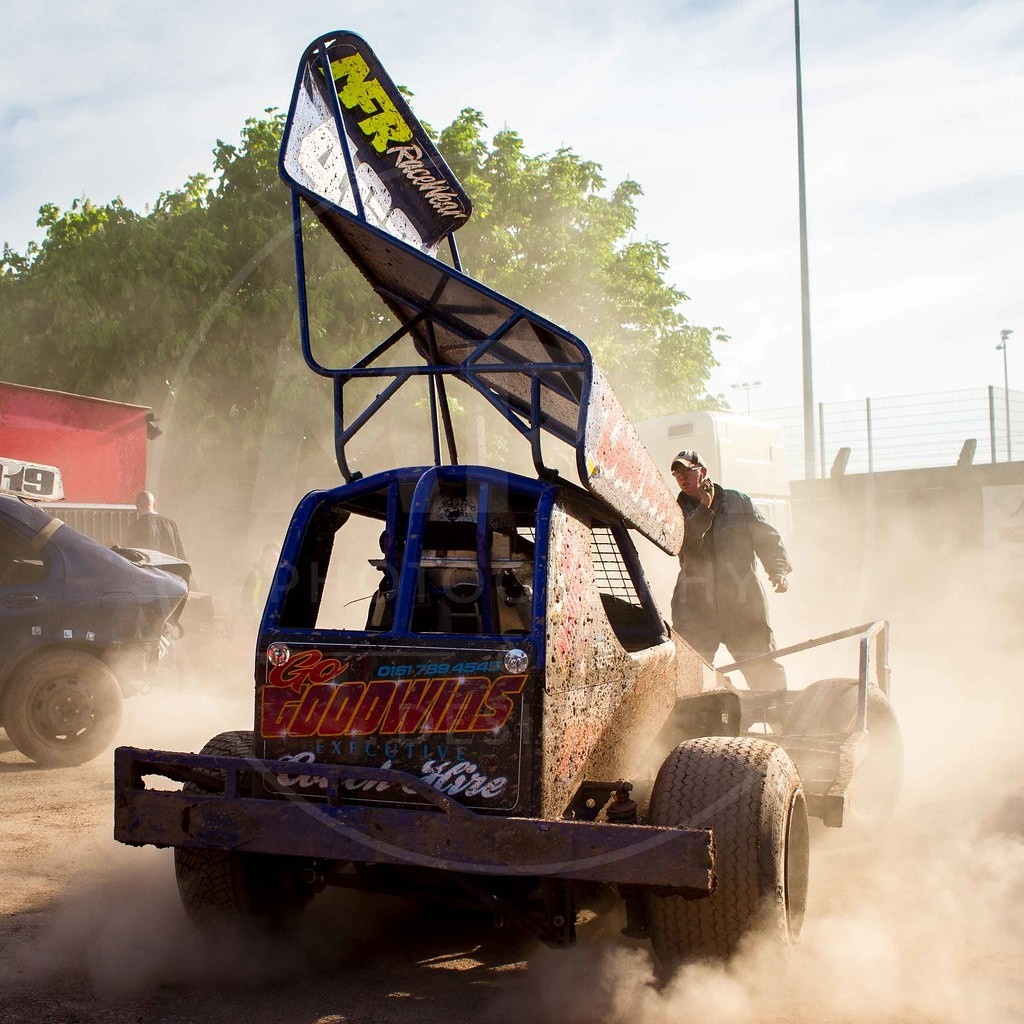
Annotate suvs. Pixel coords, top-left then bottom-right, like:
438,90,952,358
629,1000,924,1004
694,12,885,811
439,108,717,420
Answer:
1,492,189,771
115,460,904,986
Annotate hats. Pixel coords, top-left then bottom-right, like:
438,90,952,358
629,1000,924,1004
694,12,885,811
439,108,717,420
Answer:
671,450,704,469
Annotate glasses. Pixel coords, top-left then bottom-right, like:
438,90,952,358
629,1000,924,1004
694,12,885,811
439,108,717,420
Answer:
671,470,698,474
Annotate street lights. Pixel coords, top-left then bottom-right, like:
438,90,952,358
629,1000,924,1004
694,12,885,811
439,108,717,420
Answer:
992,327,1015,461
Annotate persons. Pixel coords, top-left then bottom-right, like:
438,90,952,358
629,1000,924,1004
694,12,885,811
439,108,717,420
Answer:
127,491,187,562
671,451,793,693
236,542,286,627
